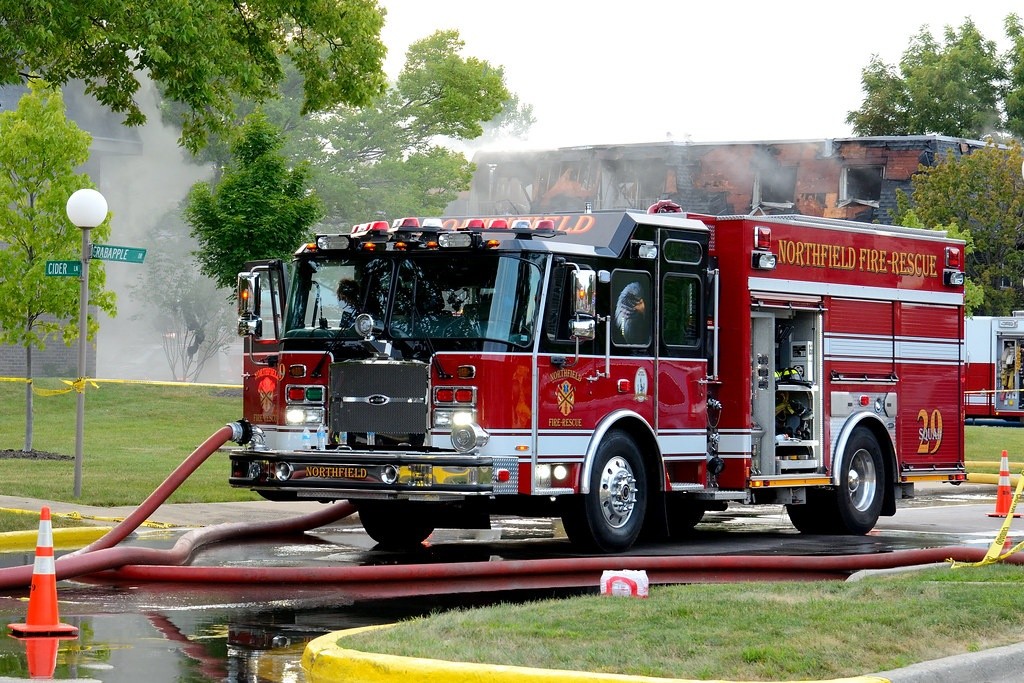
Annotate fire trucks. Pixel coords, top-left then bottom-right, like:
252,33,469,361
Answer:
221,199,968,549
964,310,1024,427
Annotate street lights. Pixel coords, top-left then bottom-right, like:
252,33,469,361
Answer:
65,185,108,492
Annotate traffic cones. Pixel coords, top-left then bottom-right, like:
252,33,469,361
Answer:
9,635,79,679
985,450,1024,519
10,505,79,631
